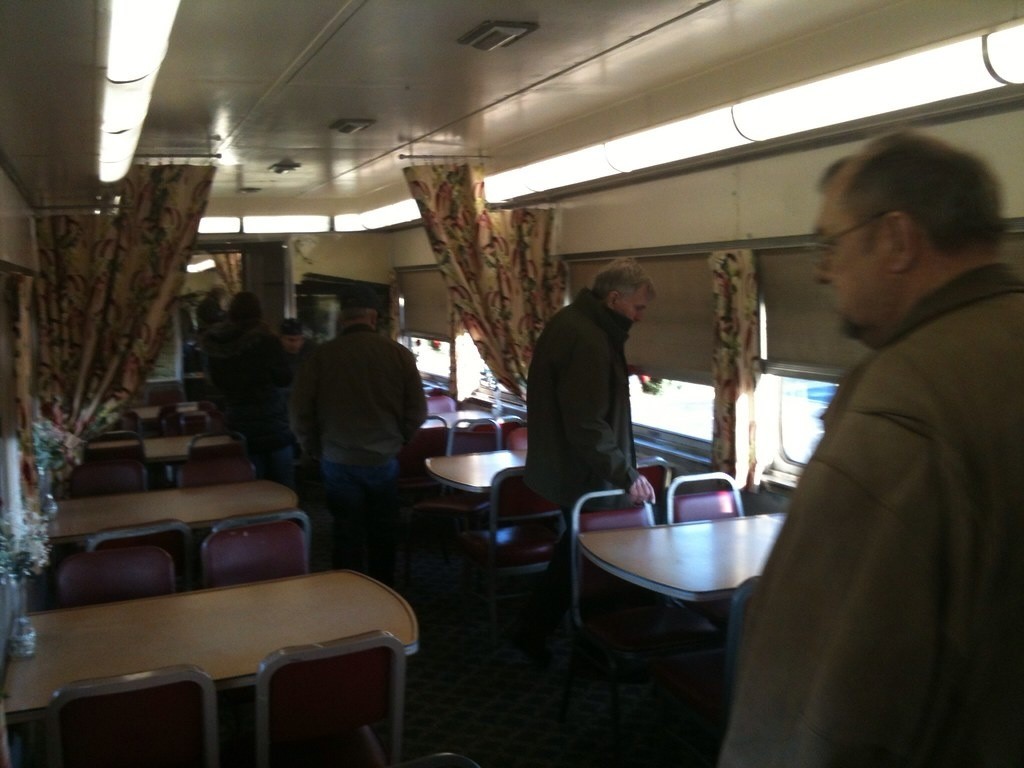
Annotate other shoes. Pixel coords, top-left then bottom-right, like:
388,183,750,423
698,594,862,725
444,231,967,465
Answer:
514,629,551,670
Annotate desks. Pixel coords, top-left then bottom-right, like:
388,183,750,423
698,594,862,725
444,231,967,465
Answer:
568,510,790,688
0,568,418,767
418,407,508,432
22,480,299,612
425,444,529,533
122,399,211,437
80,429,246,479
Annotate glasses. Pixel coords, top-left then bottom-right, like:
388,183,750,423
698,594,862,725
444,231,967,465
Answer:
808,207,898,271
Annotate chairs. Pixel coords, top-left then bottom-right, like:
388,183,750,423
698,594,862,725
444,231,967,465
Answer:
1,381,792,768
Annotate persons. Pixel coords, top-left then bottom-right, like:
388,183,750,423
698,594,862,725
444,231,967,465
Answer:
179,287,224,369
721,131,1024,768
289,290,427,590
506,258,656,683
203,290,320,488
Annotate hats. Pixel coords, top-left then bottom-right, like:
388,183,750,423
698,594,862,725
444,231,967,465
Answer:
340,286,384,320
282,319,305,337
